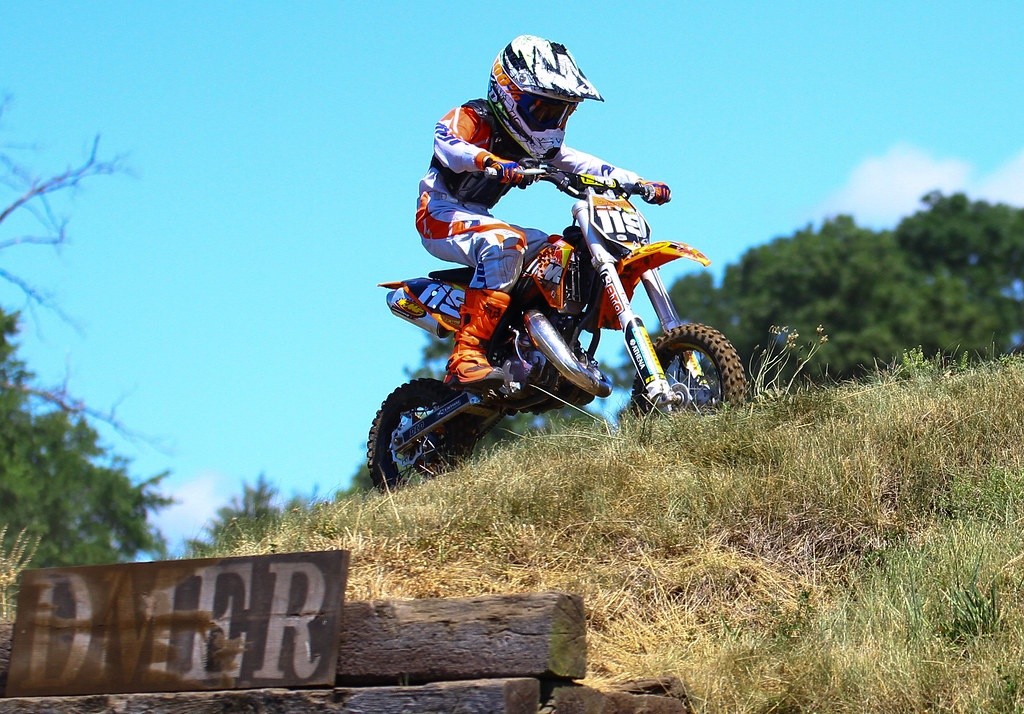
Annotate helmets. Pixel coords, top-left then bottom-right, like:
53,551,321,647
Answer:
488,34,604,161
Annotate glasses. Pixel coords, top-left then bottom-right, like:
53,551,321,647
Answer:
491,50,576,131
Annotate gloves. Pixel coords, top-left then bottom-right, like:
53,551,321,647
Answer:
486,152,524,186
635,179,671,205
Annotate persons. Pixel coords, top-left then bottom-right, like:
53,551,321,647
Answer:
417,33,672,386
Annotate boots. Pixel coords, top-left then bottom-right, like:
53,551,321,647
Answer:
443,287,511,391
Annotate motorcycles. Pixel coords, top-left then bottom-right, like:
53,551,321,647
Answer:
367,158,746,491
